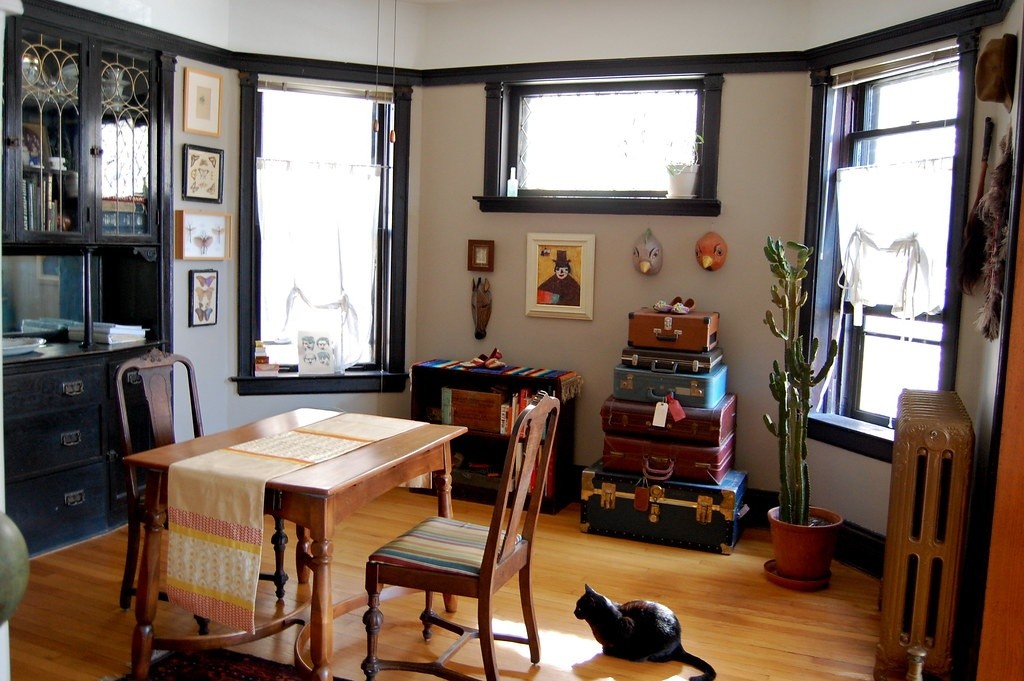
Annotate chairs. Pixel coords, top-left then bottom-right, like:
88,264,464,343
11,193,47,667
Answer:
361,387,561,681
115,345,289,635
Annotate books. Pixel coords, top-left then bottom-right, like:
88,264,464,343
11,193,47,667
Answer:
21,317,78,333
67,323,150,344
23,170,58,231
102,197,147,235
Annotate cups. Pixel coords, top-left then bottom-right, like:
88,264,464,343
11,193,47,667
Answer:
49,157,67,171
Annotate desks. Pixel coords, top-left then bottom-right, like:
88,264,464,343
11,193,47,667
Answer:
119,408,471,681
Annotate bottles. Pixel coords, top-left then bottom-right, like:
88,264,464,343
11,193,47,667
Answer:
255,341,269,364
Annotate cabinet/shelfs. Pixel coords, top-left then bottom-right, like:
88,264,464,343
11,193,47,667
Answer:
410,357,578,513
1,0,174,551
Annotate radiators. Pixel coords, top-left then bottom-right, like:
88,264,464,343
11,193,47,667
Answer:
874,390,976,681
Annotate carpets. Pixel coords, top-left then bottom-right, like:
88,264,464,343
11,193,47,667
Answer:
98,645,352,680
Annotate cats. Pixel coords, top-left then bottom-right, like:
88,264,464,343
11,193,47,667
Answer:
574,583,717,681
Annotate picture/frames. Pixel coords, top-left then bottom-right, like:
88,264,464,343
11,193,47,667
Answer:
181,143,225,203
175,208,233,261
183,66,221,137
188,269,218,328
467,239,494,273
526,232,596,321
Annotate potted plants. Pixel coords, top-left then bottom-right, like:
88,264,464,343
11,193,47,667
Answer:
761,234,845,586
666,130,704,200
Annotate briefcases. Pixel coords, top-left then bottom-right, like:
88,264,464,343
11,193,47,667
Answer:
599,394,737,446
626,306,720,353
596,431,737,487
621,346,725,375
610,362,727,410
578,458,747,557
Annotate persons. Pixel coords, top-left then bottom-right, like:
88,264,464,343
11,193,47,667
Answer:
22,133,44,166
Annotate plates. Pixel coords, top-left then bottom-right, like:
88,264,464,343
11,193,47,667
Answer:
3,338,47,356
